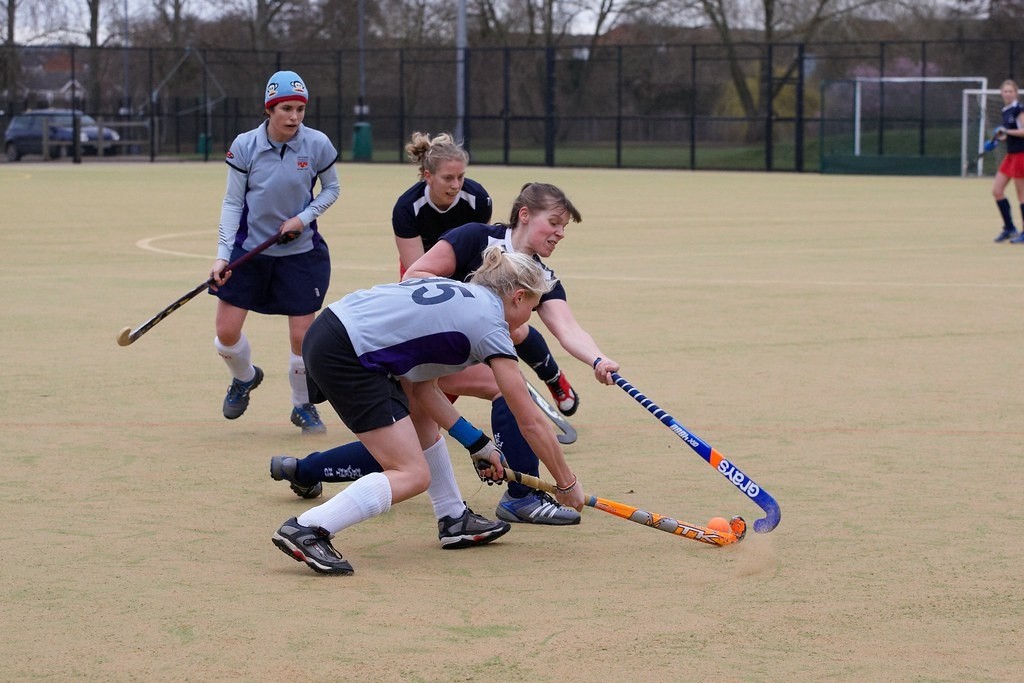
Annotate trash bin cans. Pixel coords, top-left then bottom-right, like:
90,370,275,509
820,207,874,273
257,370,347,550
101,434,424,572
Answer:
199,134,213,153
353,123,373,161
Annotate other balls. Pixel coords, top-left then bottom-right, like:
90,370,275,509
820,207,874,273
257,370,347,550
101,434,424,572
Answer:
706,517,729,533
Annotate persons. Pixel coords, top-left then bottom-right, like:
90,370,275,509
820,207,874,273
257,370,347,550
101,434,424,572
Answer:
270,242,584,576
392,132,579,417
991,80,1024,244
208,70,339,435
270,182,621,524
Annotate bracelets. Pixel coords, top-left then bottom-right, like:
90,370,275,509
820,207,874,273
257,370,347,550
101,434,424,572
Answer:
446,415,483,448
557,473,579,493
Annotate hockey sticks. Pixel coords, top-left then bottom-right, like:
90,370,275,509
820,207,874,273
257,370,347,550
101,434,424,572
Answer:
477,459,747,546
594,356,782,535
117,229,282,347
526,380,578,445
974,129,1003,164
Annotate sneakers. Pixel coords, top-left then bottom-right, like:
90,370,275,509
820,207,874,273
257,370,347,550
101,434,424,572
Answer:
290,402,328,435
1011,230,1024,244
545,369,580,417
995,226,1017,242
270,455,323,500
496,489,582,525
438,500,512,550
223,364,265,420
271,515,355,576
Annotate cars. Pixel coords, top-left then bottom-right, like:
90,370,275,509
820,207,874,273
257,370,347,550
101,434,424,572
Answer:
3,110,120,161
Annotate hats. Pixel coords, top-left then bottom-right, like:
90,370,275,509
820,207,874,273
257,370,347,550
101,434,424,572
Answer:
265,71,308,108
